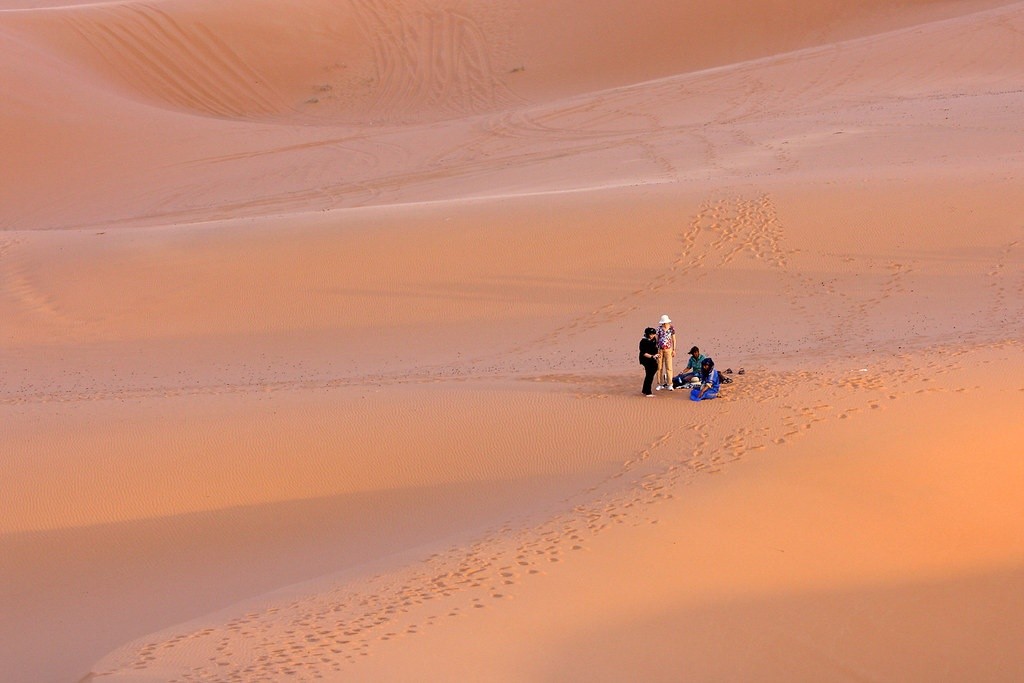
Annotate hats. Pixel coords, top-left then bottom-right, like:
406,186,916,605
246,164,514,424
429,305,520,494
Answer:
688,346,699,354
645,327,656,333
659,315,672,323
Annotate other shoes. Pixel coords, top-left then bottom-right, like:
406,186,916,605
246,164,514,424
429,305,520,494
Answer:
646,394,656,397
668,386,674,391
716,392,723,398
656,385,664,390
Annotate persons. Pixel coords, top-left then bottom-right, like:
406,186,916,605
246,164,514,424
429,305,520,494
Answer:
638,327,660,397
656,315,676,391
679,346,706,382
691,358,722,401
